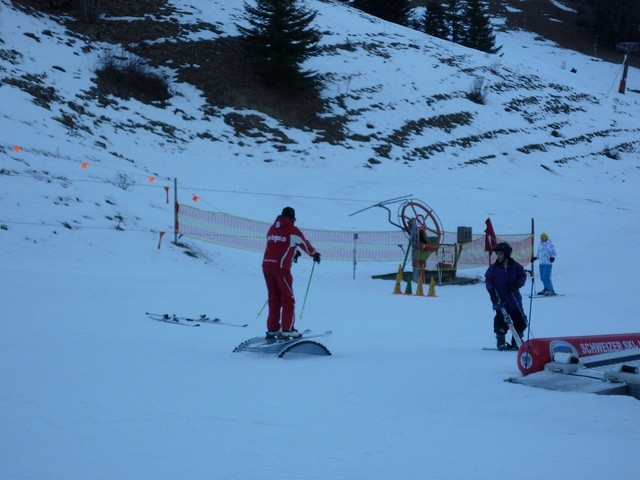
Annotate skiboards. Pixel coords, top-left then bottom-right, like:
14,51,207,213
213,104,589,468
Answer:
248,329,332,349
482,287,523,351
146,312,248,327
526,293,564,298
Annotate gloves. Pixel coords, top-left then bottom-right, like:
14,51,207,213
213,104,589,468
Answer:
313,253,320,262
507,281,519,292
531,257,537,262
293,250,301,263
550,257,555,262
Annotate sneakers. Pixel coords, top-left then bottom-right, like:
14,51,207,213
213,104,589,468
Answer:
538,289,546,294
266,330,282,339
280,329,301,339
497,342,510,350
545,291,554,295
512,340,524,349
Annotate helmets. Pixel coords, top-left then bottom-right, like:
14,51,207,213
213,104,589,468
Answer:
493,243,512,257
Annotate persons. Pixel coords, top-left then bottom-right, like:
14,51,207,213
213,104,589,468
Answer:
530,233,556,298
485,218,498,267
262,207,321,341
485,241,529,352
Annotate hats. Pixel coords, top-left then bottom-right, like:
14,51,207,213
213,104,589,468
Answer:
282,207,296,220
540,233,548,239
